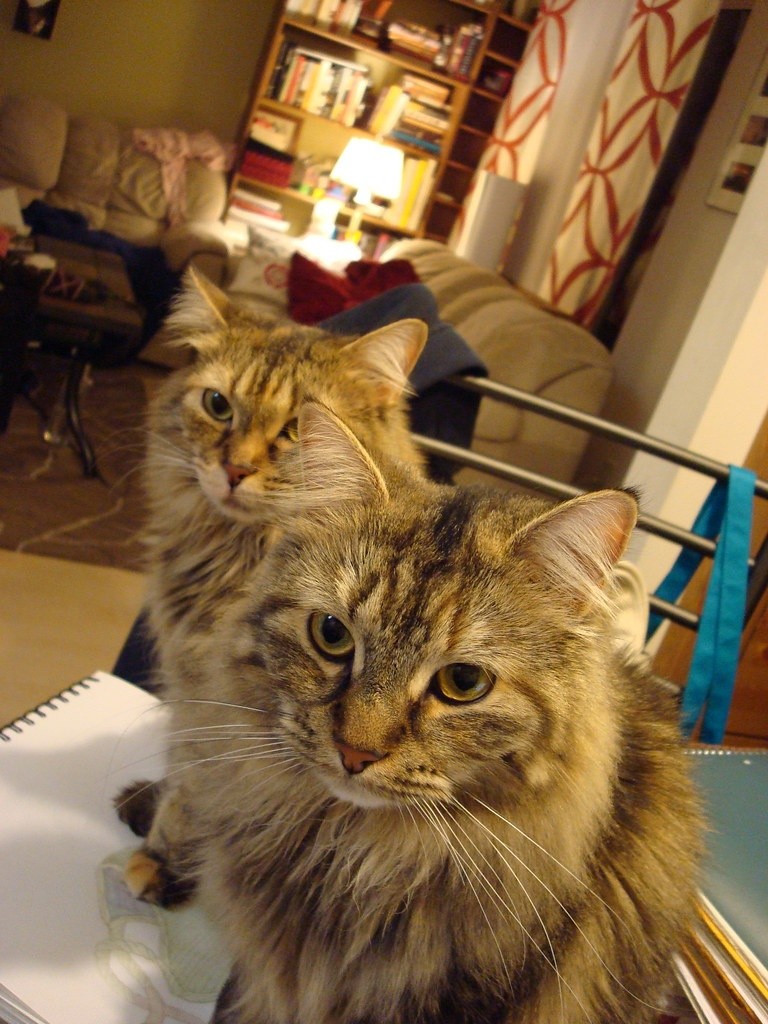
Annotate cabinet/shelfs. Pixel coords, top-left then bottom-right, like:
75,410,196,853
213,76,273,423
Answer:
215,0,540,259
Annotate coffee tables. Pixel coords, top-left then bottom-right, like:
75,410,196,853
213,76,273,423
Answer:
0,233,144,481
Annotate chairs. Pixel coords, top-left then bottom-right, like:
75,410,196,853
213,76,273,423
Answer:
403,375,767,744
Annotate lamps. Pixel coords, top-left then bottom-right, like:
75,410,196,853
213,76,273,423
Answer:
330,135,404,276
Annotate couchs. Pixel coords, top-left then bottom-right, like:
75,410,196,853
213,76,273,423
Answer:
0,93,226,374
222,235,615,499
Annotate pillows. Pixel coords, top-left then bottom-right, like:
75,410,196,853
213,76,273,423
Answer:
226,224,345,309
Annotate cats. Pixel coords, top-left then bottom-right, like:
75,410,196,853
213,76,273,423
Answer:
111,265,720,1024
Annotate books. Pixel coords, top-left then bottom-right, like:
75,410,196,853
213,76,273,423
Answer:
669,747,768,1024
225,0,484,261
0,670,237,1024
